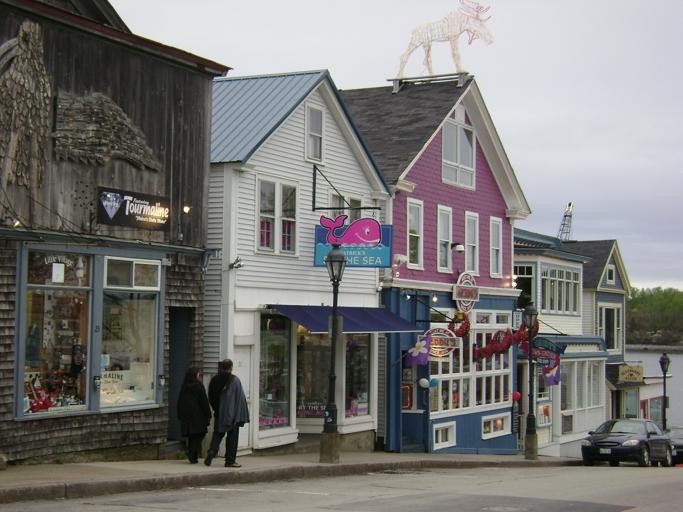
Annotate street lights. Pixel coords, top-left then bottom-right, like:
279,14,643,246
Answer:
319,239,348,463
522,300,538,460
658,349,671,433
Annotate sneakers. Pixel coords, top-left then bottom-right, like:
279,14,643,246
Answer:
203,455,214,466
224,462,241,468
186,450,198,464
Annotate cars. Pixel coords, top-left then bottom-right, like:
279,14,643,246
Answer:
662,425,683,466
259,358,307,400
580,418,672,467
24,317,140,376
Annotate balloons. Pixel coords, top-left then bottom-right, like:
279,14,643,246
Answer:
419,378,429,388
430,378,439,387
513,392,520,400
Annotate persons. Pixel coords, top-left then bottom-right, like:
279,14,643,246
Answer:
177,367,212,464
204,359,249,467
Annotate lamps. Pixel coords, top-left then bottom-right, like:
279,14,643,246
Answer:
229,256,246,272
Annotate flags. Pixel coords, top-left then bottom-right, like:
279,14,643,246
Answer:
407,333,431,365
544,364,560,385
